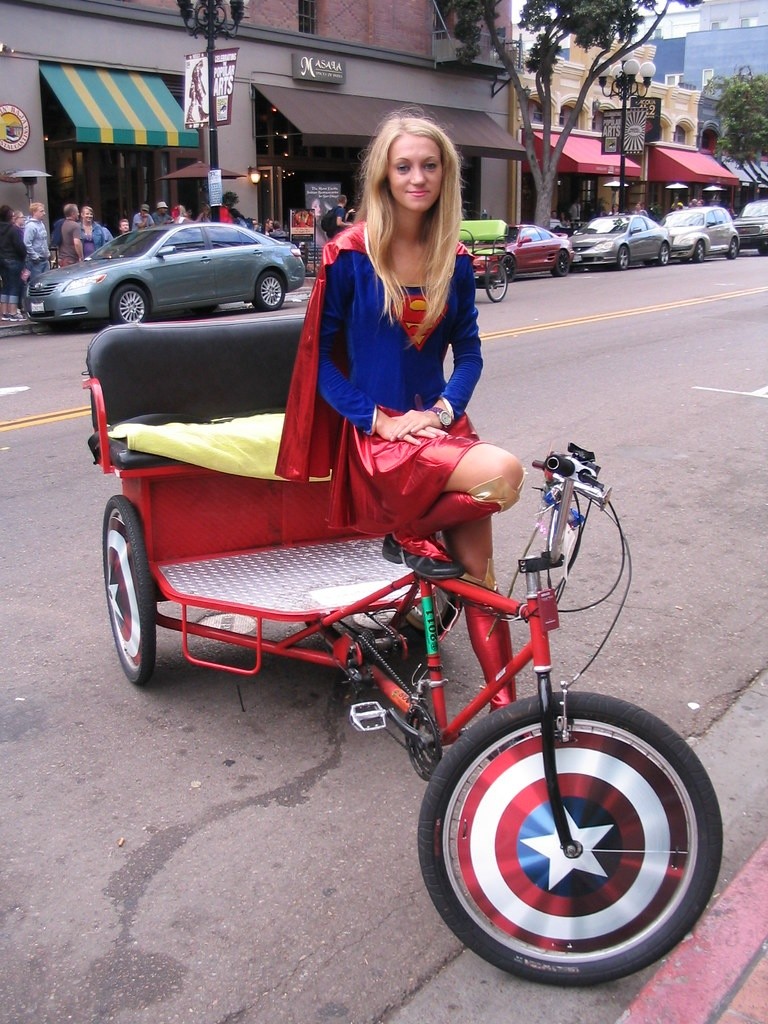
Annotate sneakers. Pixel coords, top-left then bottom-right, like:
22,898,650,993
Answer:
381,534,465,580
1,313,9,321
9,313,27,322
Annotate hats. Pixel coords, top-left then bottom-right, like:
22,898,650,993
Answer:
157,202,168,209
140,204,150,213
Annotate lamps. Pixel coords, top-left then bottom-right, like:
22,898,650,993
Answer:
592,99,601,115
248,166,261,184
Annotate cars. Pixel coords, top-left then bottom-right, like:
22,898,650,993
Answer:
23,218,305,332
472,224,575,284
658,206,741,264
567,214,672,273
732,200,768,255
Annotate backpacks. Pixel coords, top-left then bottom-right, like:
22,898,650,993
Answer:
321,206,342,233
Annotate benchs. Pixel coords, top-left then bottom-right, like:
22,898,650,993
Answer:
86,315,308,478
458,220,508,256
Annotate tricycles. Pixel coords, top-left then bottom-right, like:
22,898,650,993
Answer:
80,312,723,990
459,219,510,302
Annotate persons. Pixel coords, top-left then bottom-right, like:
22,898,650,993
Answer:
54,204,115,267
336,196,355,229
671,194,730,211
120,201,285,240
309,194,334,266
0,203,51,322
276,119,533,762
608,202,654,220
550,199,580,229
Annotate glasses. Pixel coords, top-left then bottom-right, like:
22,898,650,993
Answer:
18,216,27,219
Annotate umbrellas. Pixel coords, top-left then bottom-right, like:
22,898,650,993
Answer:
154,161,247,180
703,186,726,191
665,183,688,188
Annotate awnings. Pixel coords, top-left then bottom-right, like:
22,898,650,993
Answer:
648,147,739,185
522,131,642,177
40,64,199,147
252,82,527,161
723,160,768,184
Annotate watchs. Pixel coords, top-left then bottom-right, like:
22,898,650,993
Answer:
427,407,452,429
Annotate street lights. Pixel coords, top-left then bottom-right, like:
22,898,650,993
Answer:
173,0,252,224
591,42,656,216
